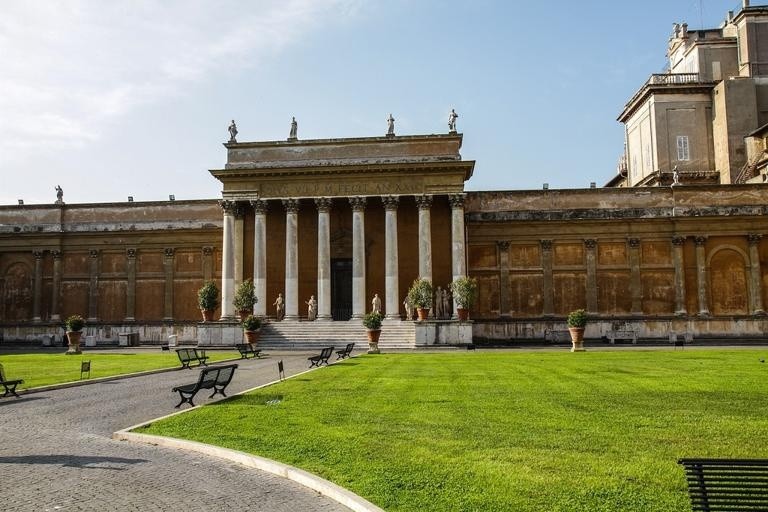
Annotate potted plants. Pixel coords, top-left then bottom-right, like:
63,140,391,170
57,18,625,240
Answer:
241,316,262,344
447,277,478,321
567,309,587,341
360,314,384,342
406,275,434,321
65,313,83,344
197,279,220,321
232,277,259,321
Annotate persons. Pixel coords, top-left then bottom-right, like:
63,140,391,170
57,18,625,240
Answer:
385,112,395,134
290,116,299,138
671,165,680,184
272,293,285,319
448,109,459,130
304,294,318,320
404,286,451,319
372,293,382,316
227,119,239,142
54,184,64,199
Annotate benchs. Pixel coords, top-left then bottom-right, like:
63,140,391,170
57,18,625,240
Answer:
676,457,767,512
236,343,263,361
0,364,24,398
175,346,211,370
306,346,333,369
335,342,356,362
170,364,237,410
605,330,638,345
